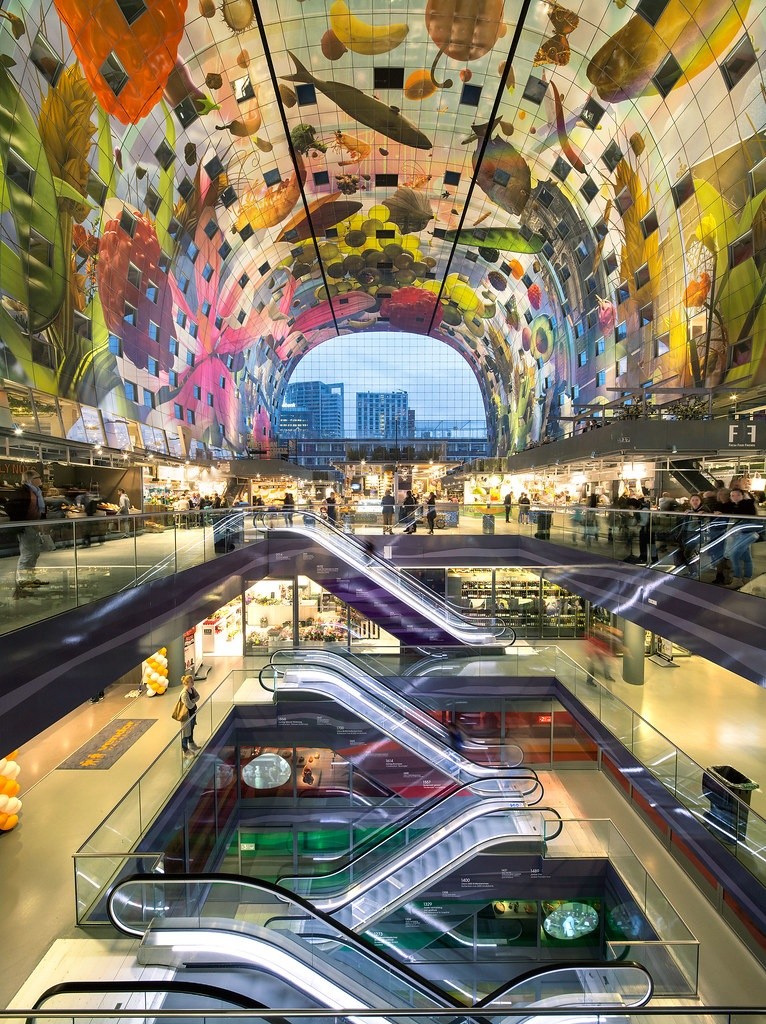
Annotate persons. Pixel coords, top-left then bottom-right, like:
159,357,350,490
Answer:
385,496,395,532
98,689,105,701
115,488,131,539
522,419,611,452
424,583,438,609
543,907,598,937
361,535,376,568
4,469,50,589
322,492,336,527
403,491,414,535
78,486,105,550
583,624,634,687
282,492,291,528
243,760,291,789
517,486,570,526
320,497,328,510
43,487,70,552
178,674,201,758
403,493,417,532
287,493,294,527
411,490,464,503
570,475,766,591
89,692,99,705
443,720,467,777
425,492,435,535
338,495,345,505
171,489,265,530
380,488,395,535
71,495,86,509
504,491,514,524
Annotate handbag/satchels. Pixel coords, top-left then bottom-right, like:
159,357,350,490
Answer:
171,692,190,722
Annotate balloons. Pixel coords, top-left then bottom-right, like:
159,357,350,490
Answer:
142,646,170,697
0,749,22,831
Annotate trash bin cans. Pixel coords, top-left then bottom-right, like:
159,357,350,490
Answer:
209,514,235,553
534,514,551,540
701,766,759,842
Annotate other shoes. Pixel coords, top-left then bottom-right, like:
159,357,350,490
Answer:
183,750,195,757
90,700,98,703
99,697,103,700
188,743,201,750
